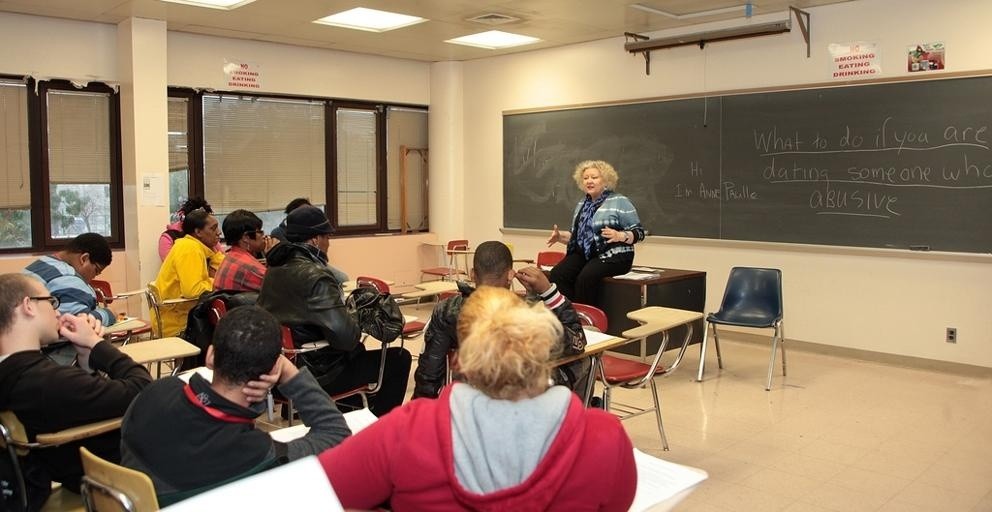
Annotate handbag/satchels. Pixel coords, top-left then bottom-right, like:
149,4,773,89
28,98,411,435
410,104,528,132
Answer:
345,288,406,342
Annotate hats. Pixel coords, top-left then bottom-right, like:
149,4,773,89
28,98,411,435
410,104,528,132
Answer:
280,204,335,235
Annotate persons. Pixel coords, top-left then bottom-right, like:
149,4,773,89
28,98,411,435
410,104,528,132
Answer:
21,195,311,331
412,243,587,402
547,161,644,300
257,205,414,416
318,288,636,511
118,307,354,509
0,271,152,495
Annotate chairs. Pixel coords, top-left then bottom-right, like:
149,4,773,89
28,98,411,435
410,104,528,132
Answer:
696,266,788,392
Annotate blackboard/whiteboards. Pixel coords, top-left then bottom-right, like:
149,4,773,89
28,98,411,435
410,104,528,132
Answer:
500,70,992,264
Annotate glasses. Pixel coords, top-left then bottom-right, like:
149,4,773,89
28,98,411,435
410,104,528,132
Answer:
94,263,102,275
30,294,60,310
244,230,263,236
